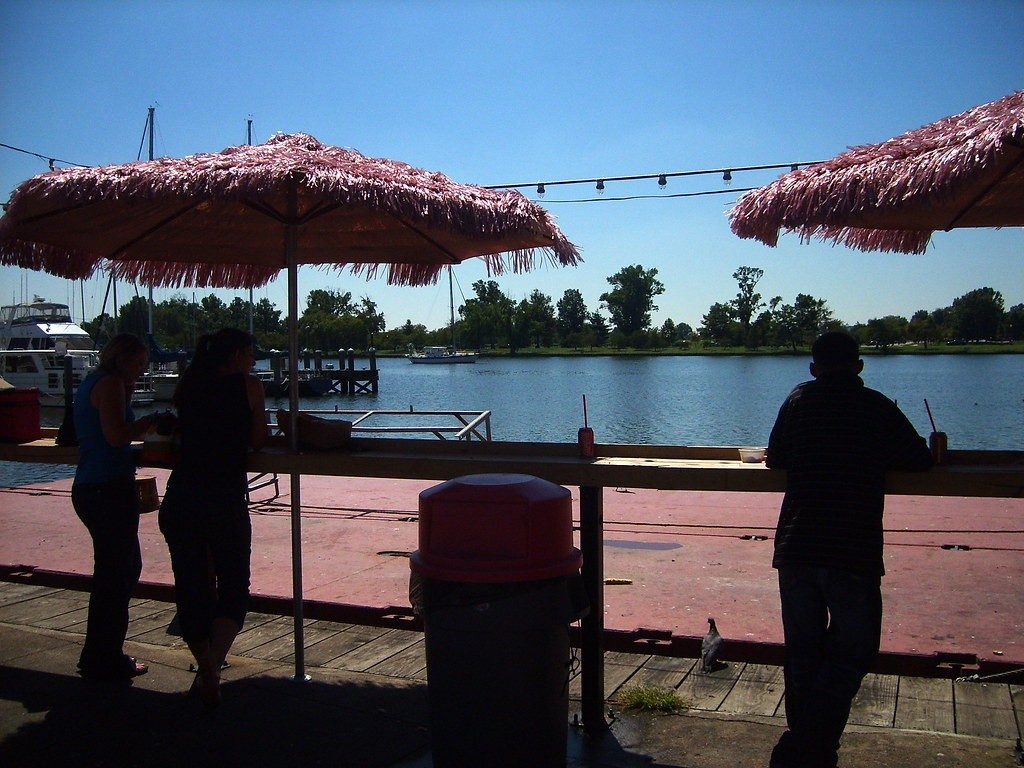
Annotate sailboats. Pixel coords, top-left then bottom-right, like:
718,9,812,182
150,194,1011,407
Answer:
404,263,486,365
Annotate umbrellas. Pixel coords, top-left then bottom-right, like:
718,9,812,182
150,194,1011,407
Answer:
1,131,579,675
729,90,1023,255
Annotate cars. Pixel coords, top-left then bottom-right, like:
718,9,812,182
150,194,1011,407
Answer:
947,339,966,346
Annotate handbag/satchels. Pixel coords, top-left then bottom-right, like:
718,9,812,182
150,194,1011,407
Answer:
141,366,184,469
55,420,78,448
275,408,352,450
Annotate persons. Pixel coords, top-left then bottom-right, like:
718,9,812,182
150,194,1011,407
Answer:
765,329,935,768
72,332,172,678
156,327,273,710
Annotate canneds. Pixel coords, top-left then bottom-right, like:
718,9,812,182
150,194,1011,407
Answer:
578,428,595,457
929,432,947,462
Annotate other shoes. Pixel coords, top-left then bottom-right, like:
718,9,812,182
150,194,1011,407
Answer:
77,656,148,679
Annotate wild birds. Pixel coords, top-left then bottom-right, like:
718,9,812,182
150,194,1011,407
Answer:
701,618,724,674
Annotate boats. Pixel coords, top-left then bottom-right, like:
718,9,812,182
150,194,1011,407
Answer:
0,257,158,409
